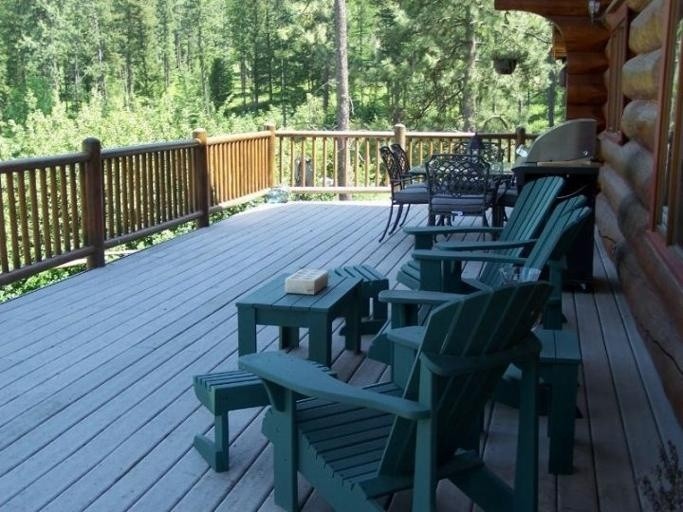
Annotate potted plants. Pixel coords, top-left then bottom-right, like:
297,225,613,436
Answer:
486,47,528,75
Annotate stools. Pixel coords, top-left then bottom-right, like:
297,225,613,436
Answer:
328,262,390,335
503,328,581,476
191,360,340,473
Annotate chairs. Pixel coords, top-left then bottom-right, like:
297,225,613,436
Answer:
382,176,590,452
245,288,546,512
376,138,519,244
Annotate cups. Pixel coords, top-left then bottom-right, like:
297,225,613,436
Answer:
515,144,531,157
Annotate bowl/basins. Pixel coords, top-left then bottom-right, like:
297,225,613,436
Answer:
499,265,542,287
